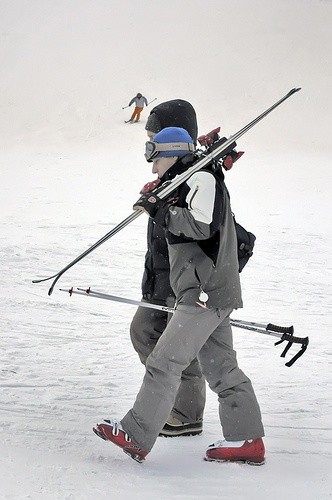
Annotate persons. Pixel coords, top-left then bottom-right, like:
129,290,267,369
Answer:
94,127,266,466
128,99,256,438
128,92,147,123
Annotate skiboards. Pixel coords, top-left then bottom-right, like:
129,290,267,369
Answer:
32,87,301,296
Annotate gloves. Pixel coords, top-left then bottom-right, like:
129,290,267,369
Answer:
132,192,163,220
129,104,130,106
146,104,147,106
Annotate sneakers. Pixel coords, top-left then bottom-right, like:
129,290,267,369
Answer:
129,119,133,121
135,120,138,122
204,436,264,465
92,419,150,463
159,414,203,437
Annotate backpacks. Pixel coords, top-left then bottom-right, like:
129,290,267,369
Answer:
231,212,256,274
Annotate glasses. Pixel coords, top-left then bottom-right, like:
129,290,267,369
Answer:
145,140,195,162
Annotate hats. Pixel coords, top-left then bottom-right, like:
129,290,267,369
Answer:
137,93,142,97
150,127,193,156
145,99,198,146
